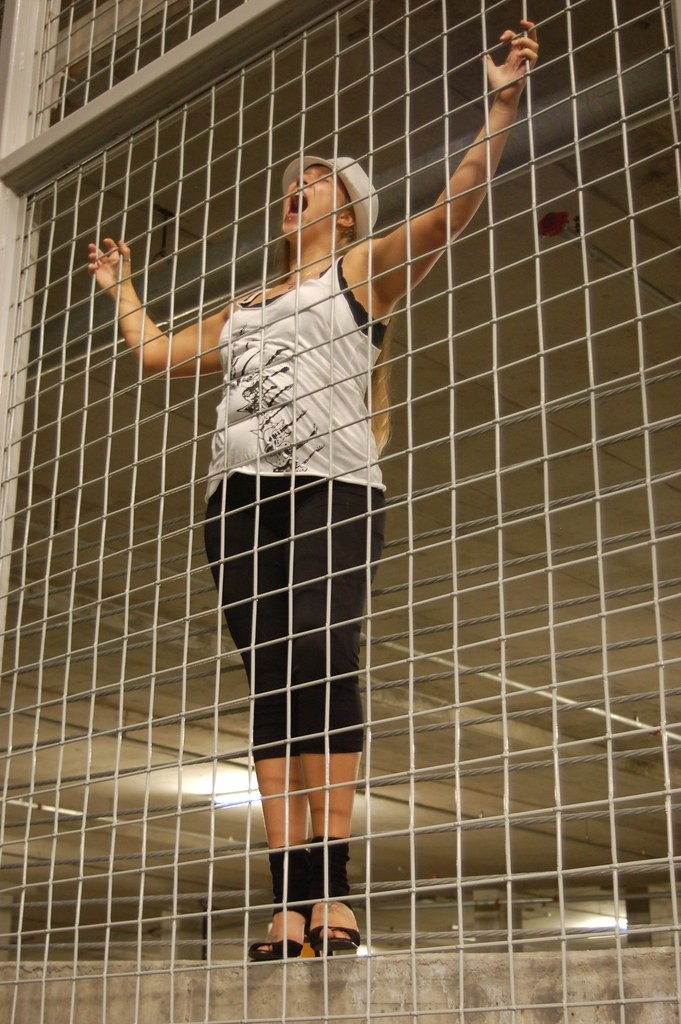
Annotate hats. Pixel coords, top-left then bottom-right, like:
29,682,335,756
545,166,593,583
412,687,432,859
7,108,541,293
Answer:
283,156,379,246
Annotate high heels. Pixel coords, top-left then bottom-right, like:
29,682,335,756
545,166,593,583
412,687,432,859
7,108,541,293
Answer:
248,899,311,960
309,889,360,957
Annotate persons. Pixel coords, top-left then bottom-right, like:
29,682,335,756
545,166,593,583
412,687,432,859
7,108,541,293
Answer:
85,19,540,956
